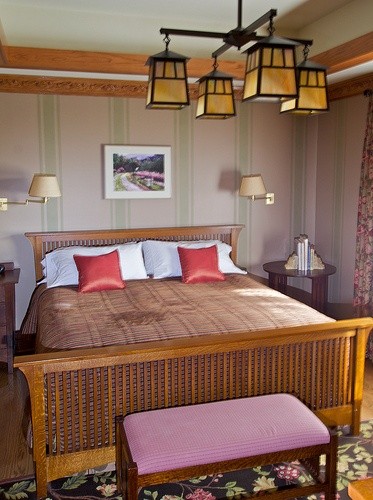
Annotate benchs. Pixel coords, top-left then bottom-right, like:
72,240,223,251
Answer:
115,393,337,500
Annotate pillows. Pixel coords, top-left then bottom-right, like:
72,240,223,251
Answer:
73,249,126,294
177,245,225,284
36,242,149,289
143,240,247,280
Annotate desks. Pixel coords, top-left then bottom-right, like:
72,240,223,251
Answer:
263,261,336,314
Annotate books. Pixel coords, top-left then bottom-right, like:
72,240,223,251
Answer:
294,234,315,271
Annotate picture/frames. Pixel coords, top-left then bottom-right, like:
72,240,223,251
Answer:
104,145,172,199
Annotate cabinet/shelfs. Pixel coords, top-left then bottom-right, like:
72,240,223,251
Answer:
0,266,20,374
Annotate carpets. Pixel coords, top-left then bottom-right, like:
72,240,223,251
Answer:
0,419,373,500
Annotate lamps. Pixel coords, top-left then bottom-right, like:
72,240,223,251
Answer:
240,175,274,205
0,174,61,211
145,0,331,120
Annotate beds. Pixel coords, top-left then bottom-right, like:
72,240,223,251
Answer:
13,225,373,500
